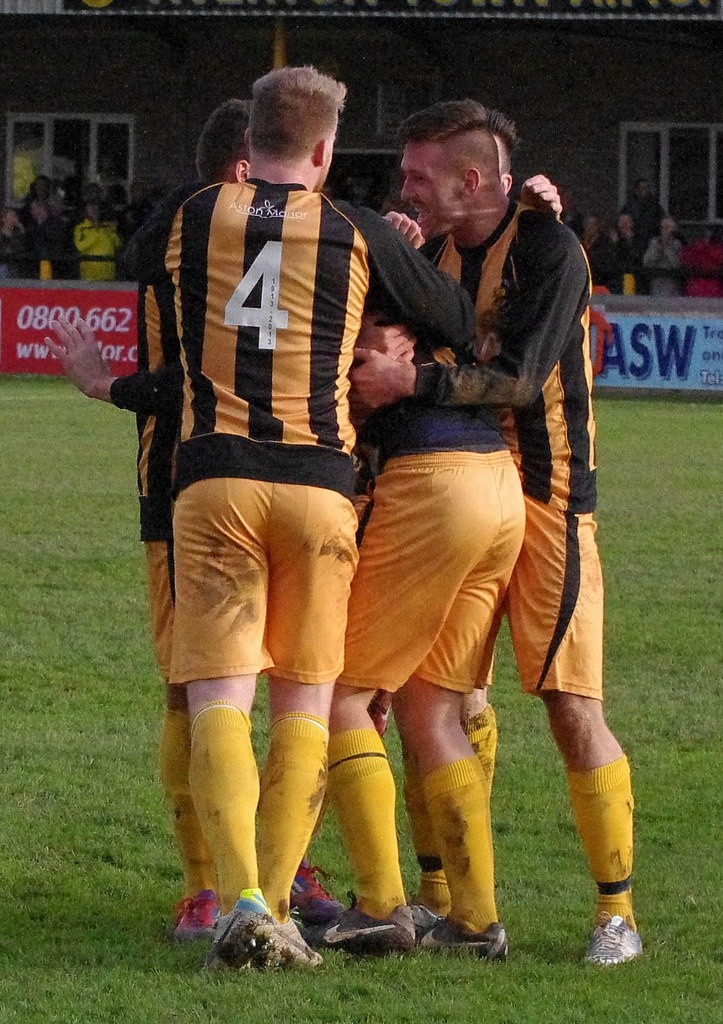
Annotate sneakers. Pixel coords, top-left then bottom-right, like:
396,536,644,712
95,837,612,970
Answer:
289,861,344,924
416,915,508,962
202,888,324,967
585,911,643,965
174,889,221,938
406,894,446,947
300,905,416,954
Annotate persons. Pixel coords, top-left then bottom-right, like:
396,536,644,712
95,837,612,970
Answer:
561,177,677,294
45,67,564,970
348,99,645,969
0,173,145,283
